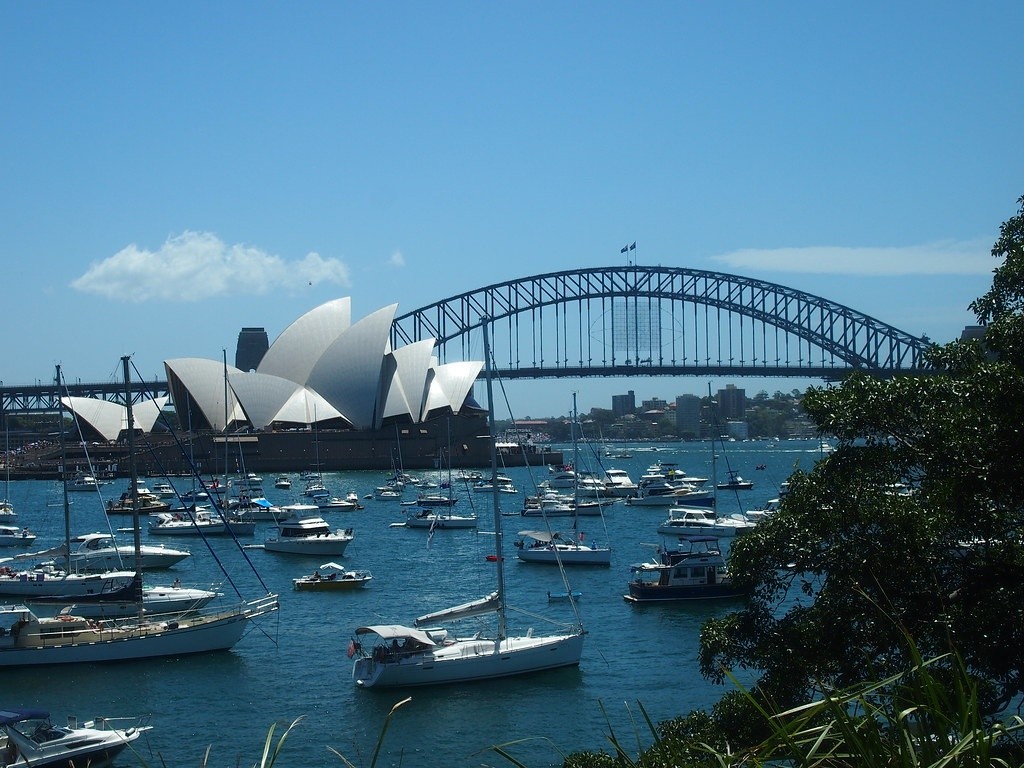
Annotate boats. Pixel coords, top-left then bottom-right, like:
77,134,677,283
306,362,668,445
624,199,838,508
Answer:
502,512,519,517
274,477,292,489
627,461,715,507
815,443,838,453
486,556,505,561
731,478,915,523
453,470,483,483
293,562,374,589
401,501,416,506
538,465,638,496
414,482,438,488
728,436,838,441
718,471,754,490
623,535,751,603
547,593,582,602
264,503,354,556
0,708,153,768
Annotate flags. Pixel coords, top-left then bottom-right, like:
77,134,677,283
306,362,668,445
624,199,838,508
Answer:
630,242,636,250
621,245,628,253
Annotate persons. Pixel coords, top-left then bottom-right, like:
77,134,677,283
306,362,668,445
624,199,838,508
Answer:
668,469,674,480
564,463,573,471
735,473,738,477
108,497,114,510
388,640,400,649
9,439,53,466
314,570,321,578
580,532,584,545
592,540,597,550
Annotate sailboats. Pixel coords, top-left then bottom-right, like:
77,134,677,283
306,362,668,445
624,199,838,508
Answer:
299,402,364,513
348,314,589,690
655,381,758,537
522,410,624,516
406,417,480,528
417,447,459,507
514,392,612,566
373,417,420,501
0,354,282,666
594,425,633,461
474,436,519,494
